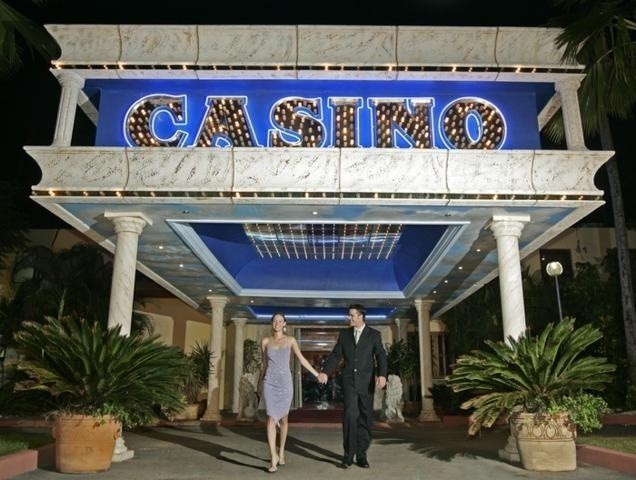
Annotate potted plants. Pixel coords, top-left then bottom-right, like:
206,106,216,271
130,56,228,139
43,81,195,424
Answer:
5,312,217,475
241,337,265,423
438,314,620,474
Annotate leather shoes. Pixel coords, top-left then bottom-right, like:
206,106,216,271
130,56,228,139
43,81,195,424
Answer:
341,455,354,469
356,458,370,468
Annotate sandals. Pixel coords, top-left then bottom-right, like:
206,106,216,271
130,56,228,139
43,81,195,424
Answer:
268,453,280,474
277,453,286,467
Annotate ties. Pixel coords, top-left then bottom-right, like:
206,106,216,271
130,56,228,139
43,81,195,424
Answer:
354,330,360,346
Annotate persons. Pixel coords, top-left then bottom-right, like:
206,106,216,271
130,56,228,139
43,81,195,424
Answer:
255,311,325,474
317,303,390,470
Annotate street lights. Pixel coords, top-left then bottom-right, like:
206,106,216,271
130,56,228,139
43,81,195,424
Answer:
545,257,564,324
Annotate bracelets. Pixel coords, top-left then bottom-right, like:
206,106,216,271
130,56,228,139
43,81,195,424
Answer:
258,382,262,385
